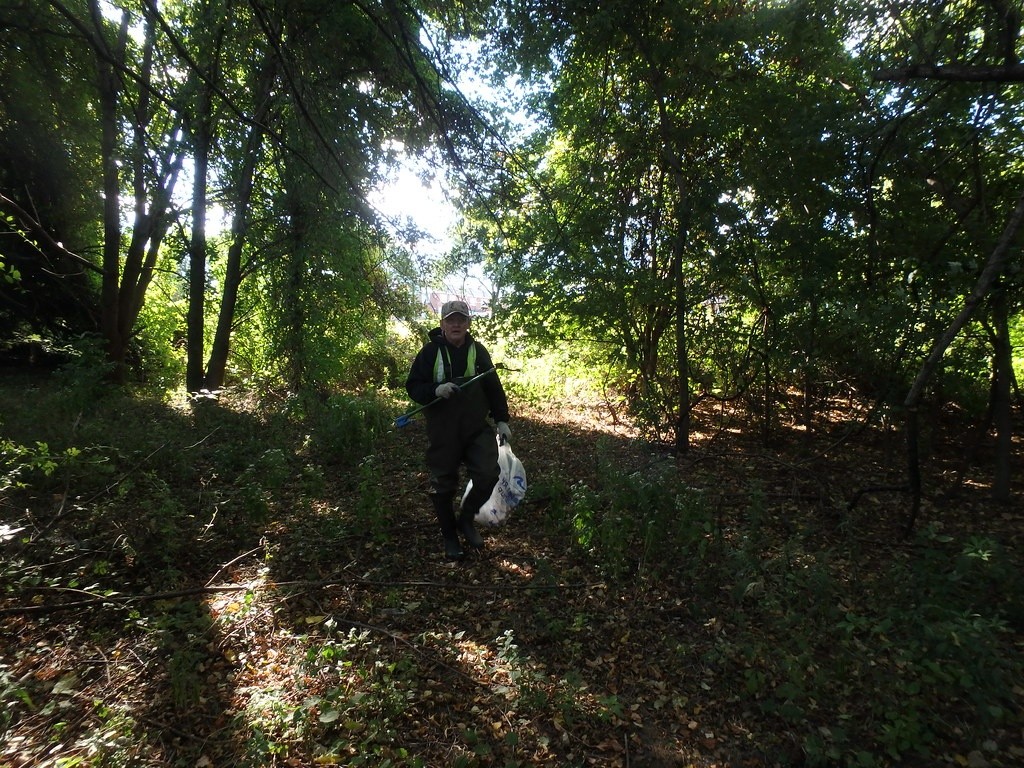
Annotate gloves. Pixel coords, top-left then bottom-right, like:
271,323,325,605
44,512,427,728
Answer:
498,422,513,447
435,382,460,399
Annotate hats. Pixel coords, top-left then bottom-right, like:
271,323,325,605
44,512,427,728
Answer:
441,301,470,319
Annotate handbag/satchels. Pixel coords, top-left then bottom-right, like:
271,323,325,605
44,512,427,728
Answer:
474,434,527,529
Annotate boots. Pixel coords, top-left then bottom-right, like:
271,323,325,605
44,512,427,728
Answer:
443,528,465,560
457,513,484,547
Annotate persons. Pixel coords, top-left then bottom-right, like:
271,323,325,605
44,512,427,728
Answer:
406,301,513,560
